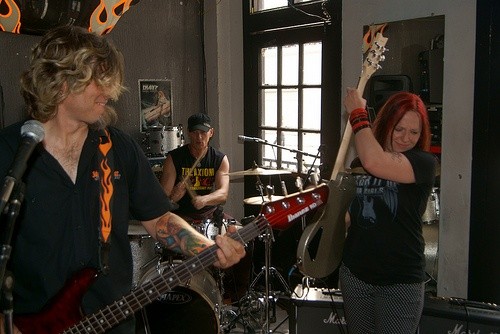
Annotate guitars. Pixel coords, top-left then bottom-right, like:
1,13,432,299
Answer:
295,31,389,280
7,171,331,334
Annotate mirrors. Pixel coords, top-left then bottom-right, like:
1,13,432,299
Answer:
363,15,445,297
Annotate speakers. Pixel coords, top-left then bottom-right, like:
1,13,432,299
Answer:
370,74,414,117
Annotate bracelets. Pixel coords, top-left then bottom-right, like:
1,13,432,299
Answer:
349,108,372,135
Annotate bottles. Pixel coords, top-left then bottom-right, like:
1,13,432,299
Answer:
268,284,276,323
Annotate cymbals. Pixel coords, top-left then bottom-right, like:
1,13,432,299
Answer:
220,168,291,176
244,195,282,205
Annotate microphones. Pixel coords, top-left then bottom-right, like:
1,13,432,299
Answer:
238,134,267,145
1,119,46,204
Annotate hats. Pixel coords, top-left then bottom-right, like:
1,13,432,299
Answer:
188,112,213,133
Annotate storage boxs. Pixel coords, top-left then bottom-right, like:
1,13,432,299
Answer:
288,285,344,334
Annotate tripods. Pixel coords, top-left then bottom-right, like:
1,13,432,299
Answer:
228,223,292,323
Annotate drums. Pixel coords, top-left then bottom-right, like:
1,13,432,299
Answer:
135,259,221,334
419,187,439,223
125,219,159,292
192,217,236,242
144,122,184,156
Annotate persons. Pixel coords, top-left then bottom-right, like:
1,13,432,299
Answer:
0,26,245,334
340,87,437,334
160,113,250,308
